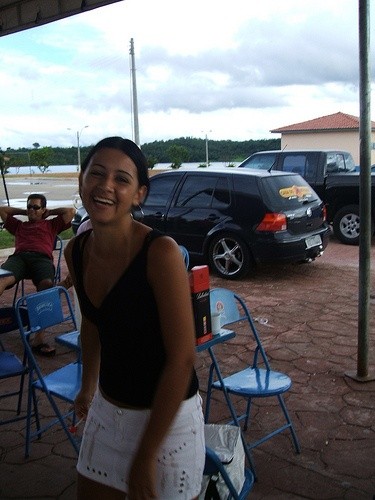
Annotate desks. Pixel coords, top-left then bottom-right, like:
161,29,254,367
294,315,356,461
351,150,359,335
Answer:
54,326,236,353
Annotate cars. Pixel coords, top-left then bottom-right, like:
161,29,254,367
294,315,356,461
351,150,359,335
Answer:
70,168,334,280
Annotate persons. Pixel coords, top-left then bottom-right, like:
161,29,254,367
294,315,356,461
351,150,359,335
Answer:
57,219,93,290
0,194,75,358
63,136,206,500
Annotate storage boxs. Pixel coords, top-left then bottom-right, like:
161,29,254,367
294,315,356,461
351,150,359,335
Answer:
187,265,212,346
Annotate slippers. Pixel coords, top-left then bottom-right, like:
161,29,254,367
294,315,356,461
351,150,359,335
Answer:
30,342,57,356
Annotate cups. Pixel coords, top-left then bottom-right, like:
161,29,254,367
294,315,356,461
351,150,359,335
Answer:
211,313,226,335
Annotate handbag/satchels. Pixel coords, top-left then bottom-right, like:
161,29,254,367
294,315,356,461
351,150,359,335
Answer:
200,422,246,500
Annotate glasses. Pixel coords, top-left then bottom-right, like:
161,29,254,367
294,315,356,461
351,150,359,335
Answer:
26,204,42,211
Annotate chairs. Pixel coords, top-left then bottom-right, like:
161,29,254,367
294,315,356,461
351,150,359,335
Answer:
201,446,255,500
0,234,82,459
205,288,302,481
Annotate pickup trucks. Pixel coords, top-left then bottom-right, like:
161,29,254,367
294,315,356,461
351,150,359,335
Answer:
238,149,375,245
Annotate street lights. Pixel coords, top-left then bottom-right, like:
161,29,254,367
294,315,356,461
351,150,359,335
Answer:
67,126,88,173
202,129,213,168
